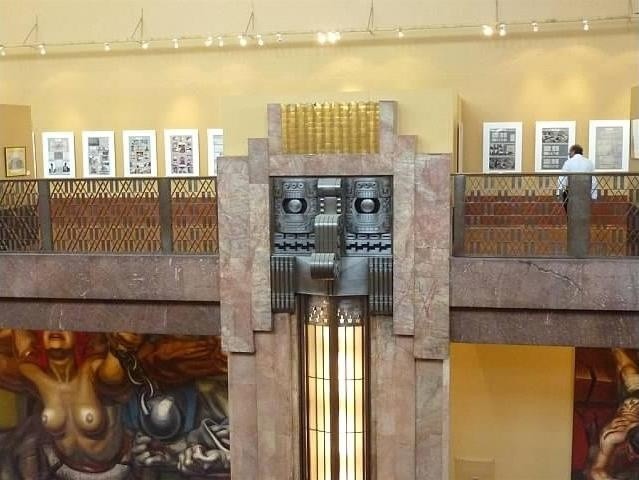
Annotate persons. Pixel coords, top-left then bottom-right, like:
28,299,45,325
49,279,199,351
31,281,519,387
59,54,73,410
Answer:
555,144,598,222
11,327,147,480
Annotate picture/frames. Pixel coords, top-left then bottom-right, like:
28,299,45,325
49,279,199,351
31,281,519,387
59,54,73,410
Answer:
5,147,26,177
483,120,631,173
42,129,224,178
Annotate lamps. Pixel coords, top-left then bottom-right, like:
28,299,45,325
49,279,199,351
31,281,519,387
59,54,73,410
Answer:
0,16,639,57
